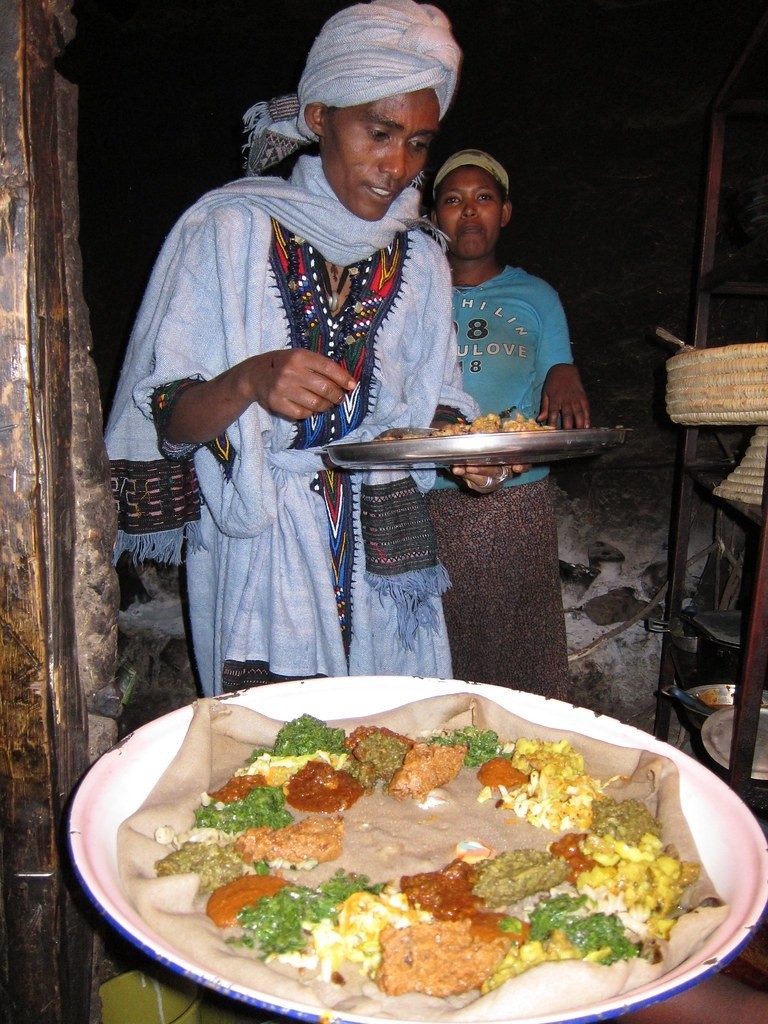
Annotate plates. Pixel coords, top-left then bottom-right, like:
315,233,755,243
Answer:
702,705,768,780
68,675,768,1024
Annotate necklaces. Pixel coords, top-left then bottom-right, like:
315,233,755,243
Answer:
288,231,364,363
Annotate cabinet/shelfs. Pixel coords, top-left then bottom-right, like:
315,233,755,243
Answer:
653,13,767,827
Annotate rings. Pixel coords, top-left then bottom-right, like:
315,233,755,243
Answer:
496,466,510,481
479,476,493,488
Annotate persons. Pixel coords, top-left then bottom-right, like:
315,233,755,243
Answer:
422,150,592,703
132,1,532,700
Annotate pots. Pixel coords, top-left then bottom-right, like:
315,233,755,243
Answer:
656,684,768,737
643,613,706,654
